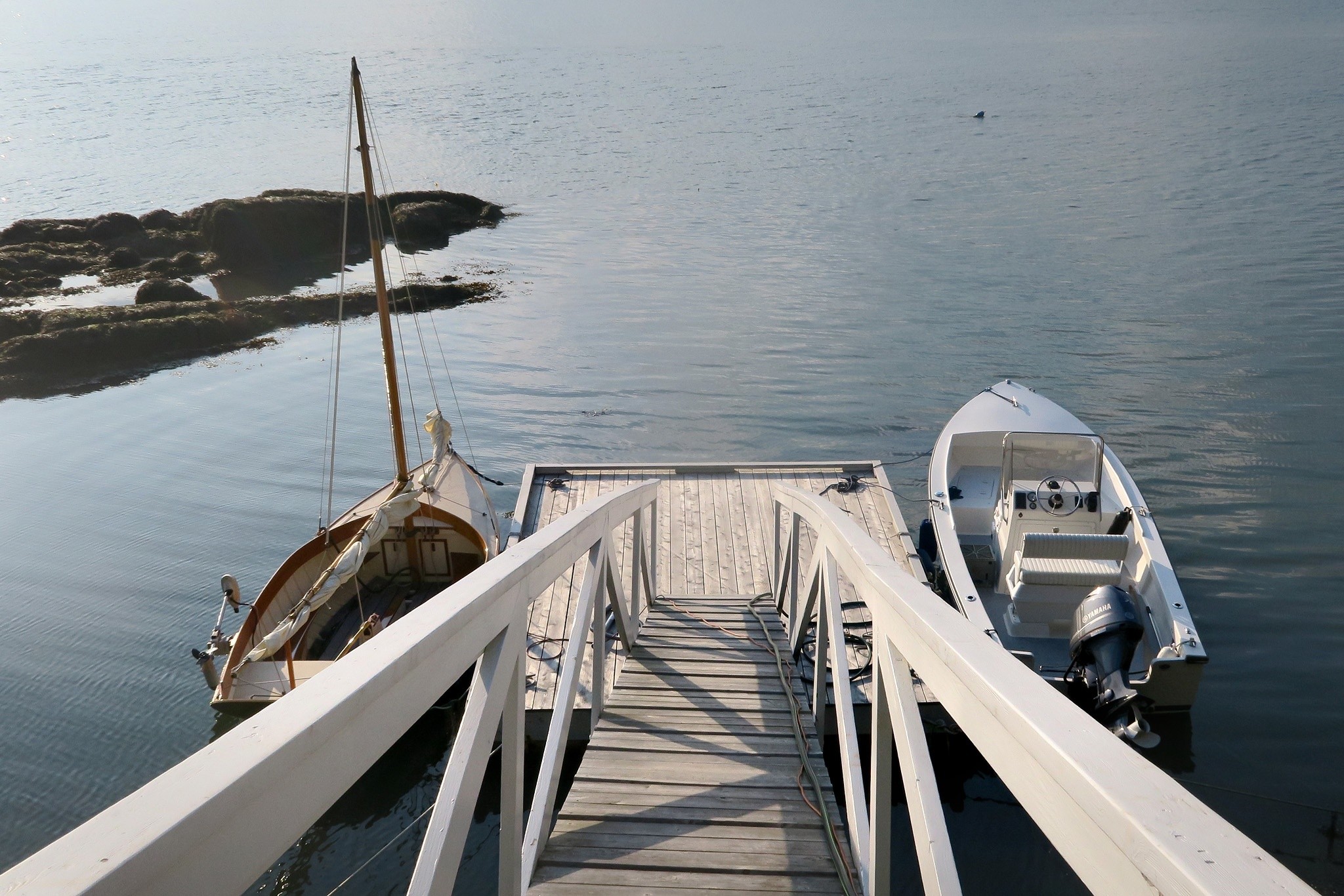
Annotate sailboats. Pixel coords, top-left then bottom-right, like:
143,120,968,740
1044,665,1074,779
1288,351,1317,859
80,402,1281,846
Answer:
192,55,501,717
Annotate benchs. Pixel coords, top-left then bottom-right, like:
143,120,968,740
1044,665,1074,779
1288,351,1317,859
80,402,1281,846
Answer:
263,550,380,662
1013,531,1131,587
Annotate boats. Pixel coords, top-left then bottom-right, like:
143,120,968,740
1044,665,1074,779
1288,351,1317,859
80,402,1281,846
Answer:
913,379,1212,714
